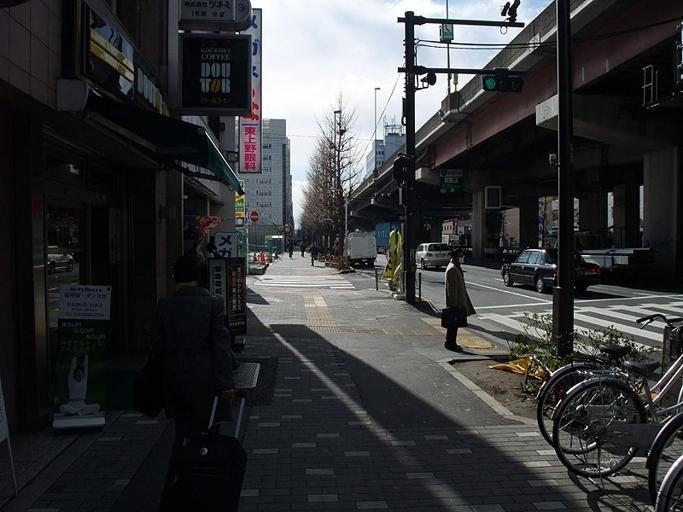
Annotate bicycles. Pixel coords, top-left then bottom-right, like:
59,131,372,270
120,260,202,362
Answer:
533,313,683,511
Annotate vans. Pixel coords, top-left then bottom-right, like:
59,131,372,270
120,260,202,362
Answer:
549,225,558,234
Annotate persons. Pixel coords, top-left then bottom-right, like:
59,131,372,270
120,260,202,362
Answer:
286,241,293,257
299,242,305,255
443,248,475,351
146,243,235,469
308,243,316,266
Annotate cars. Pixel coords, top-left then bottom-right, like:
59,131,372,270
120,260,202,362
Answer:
46,245,74,274
499,247,603,297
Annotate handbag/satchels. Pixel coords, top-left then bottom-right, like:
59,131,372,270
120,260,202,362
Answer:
439,305,469,329
131,356,174,419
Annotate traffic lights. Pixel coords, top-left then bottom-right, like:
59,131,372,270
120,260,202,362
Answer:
480,74,525,95
390,156,414,183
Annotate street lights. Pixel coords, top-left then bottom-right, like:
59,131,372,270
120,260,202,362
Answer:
331,110,345,193
374,85,381,178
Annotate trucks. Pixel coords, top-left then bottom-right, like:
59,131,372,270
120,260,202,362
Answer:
343,231,379,270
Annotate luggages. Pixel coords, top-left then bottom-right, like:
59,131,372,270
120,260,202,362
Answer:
155,388,250,512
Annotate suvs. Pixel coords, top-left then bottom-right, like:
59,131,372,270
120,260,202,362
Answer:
415,242,455,272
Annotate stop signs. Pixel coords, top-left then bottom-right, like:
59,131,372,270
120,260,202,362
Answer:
250,211,259,223
